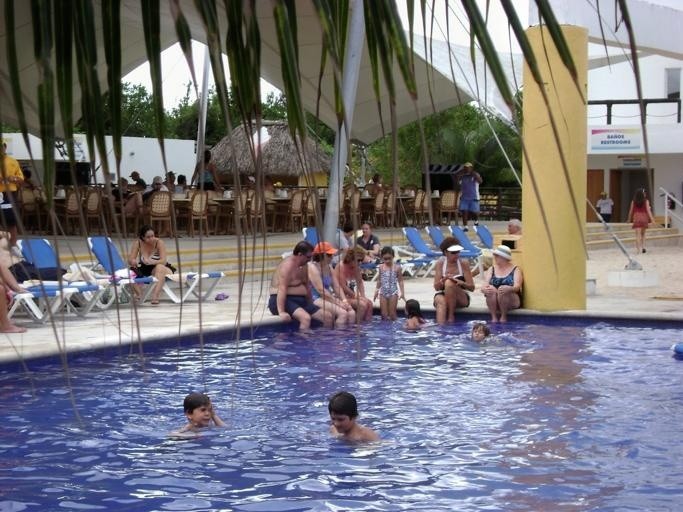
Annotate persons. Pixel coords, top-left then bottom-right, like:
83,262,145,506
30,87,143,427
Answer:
470,323,490,344
126,225,176,304
457,163,483,232
265,219,425,332
242,175,274,193
0,222,81,309
627,188,657,255
470,218,523,278
363,174,386,197
0,260,30,333
105,150,223,240
163,393,228,436
329,391,380,443
10,169,37,226
595,191,614,224
0,140,25,229
433,236,475,324
481,245,522,321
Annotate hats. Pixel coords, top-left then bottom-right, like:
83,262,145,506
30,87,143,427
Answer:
248,177,256,183
273,182,282,187
493,245,512,261
446,244,464,252
464,161,472,167
312,241,339,255
166,172,176,176
154,176,162,184
129,172,139,177
600,191,607,195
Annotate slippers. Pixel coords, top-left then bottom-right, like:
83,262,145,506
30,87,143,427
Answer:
216,294,229,300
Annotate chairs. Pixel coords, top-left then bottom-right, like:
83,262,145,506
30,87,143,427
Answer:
6,237,225,326
303,226,492,282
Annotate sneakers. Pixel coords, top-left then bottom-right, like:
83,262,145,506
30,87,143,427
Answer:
464,221,478,232
